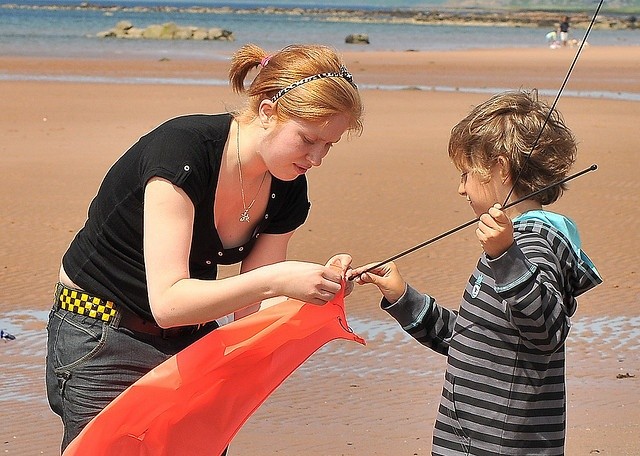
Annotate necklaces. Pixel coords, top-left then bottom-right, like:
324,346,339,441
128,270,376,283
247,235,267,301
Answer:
237,121,268,223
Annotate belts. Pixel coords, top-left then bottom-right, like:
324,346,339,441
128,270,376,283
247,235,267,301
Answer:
51,281,207,338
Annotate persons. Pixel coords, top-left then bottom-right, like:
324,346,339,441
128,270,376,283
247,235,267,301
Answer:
46,43,365,456
546,30,557,47
351,83,603,456
559,17,570,46
